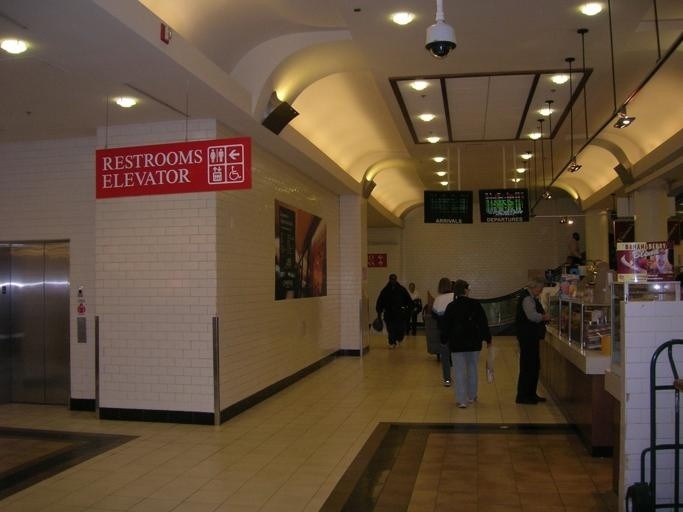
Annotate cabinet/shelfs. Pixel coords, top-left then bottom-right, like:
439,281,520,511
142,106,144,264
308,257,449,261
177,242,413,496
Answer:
607,279,683,379
547,294,615,354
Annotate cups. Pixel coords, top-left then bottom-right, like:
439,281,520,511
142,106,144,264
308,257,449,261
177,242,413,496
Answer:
600,334,610,357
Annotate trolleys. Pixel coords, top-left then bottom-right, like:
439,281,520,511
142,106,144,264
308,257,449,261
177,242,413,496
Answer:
626,338,682,511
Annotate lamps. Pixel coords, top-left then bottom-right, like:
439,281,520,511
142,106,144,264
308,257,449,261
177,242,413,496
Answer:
524,1,683,221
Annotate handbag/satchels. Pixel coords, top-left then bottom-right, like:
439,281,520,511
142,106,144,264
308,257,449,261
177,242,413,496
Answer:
485,344,494,384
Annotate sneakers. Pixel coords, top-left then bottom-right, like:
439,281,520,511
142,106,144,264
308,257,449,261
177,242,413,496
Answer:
458,402,466,408
466,398,473,402
444,379,451,386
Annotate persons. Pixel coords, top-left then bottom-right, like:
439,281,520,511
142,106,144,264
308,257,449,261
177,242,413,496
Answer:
429,277,456,386
440,279,494,408
567,231,582,261
405,281,419,334
515,277,553,403
376,274,412,348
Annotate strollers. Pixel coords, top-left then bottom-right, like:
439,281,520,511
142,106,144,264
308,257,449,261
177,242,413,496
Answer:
421,304,450,363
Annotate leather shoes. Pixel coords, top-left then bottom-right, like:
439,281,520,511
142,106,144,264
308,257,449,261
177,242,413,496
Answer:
535,395,545,401
516,400,537,404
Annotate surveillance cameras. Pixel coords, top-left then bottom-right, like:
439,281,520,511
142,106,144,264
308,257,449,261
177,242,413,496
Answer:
424,23,457,60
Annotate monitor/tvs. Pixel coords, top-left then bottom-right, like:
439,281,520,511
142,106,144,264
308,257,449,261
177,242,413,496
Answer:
424,190,473,224
479,188,529,223
